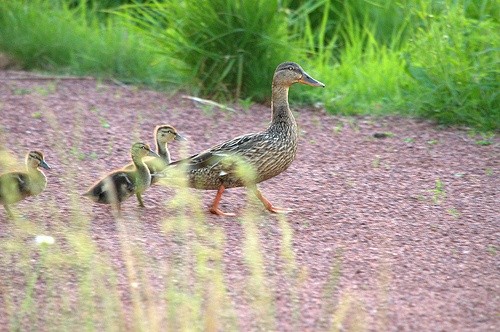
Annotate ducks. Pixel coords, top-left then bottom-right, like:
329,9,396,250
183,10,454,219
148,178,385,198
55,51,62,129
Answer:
153,62,326,220
0,150,52,218
121,123,188,208
79,141,160,215
